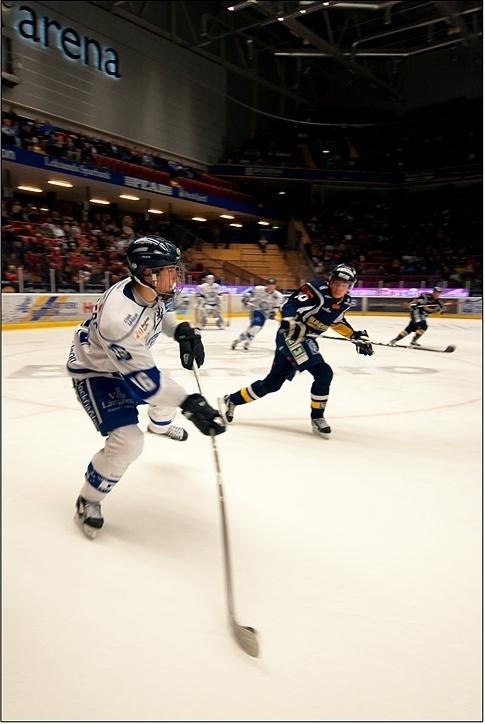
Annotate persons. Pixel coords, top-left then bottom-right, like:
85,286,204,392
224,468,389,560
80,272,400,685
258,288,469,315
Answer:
195,272,228,328
68,234,204,530
2,117,192,292
231,278,284,351
391,287,444,346
304,208,483,286
218,117,483,169
216,262,373,435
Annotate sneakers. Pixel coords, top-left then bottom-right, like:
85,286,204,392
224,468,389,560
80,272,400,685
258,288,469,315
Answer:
311,412,331,433
223,395,235,422
412,341,421,346
77,495,103,529
147,424,187,441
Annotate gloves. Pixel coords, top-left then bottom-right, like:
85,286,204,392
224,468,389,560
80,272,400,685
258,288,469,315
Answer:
350,330,373,356
180,393,225,436
174,322,204,370
409,304,418,312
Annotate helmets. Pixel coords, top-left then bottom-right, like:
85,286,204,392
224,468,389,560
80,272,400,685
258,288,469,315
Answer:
434,286,443,293
329,263,357,294
126,236,184,300
